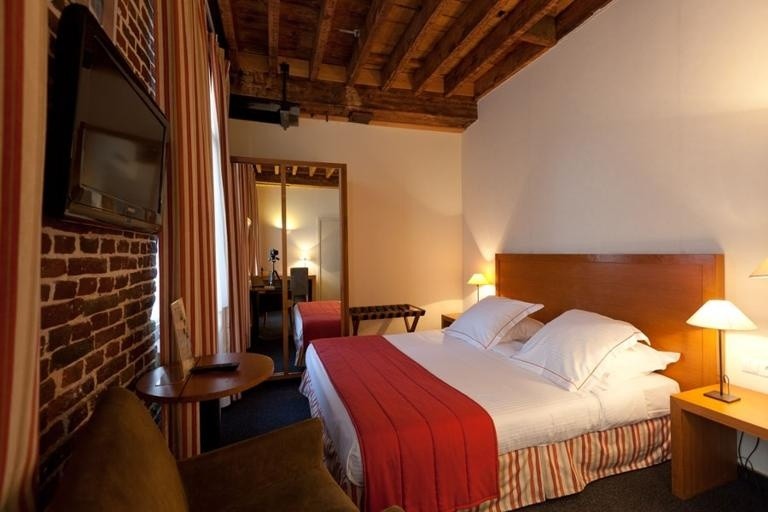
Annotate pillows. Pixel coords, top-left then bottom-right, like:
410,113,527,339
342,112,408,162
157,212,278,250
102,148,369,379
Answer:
440,295,681,396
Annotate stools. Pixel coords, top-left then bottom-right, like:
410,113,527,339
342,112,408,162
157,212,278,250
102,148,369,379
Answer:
350,305,426,336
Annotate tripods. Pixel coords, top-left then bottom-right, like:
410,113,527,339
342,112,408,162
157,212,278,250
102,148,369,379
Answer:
268,262,283,286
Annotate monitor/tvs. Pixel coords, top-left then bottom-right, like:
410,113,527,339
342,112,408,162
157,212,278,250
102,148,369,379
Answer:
49,2,170,238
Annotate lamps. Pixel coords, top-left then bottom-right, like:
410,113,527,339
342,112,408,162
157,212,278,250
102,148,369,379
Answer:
467,273,488,302
684,299,758,403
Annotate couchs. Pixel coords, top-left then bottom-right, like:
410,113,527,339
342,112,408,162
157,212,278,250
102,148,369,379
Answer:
41,386,405,511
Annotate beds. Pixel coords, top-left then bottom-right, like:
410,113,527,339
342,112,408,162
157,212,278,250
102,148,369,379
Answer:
298,253,725,512
292,300,341,368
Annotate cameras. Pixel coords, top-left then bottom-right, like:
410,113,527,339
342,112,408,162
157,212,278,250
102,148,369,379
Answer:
268,248,281,263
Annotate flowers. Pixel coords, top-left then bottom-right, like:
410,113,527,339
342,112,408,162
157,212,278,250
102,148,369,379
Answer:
267,248,280,270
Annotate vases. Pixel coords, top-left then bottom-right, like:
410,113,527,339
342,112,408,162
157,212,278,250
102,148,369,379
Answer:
269,271,276,286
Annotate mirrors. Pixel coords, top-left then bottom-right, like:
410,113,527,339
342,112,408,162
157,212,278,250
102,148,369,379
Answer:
231,156,349,382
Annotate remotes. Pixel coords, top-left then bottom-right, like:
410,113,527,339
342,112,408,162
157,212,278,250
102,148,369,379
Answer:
188,360,241,377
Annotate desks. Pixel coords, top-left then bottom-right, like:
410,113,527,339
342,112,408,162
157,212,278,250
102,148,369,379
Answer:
134,352,275,461
251,287,289,340
262,275,317,303
441,312,463,329
670,383,768,502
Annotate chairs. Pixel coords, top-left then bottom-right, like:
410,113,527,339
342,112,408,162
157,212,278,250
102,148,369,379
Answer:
288,267,309,327
